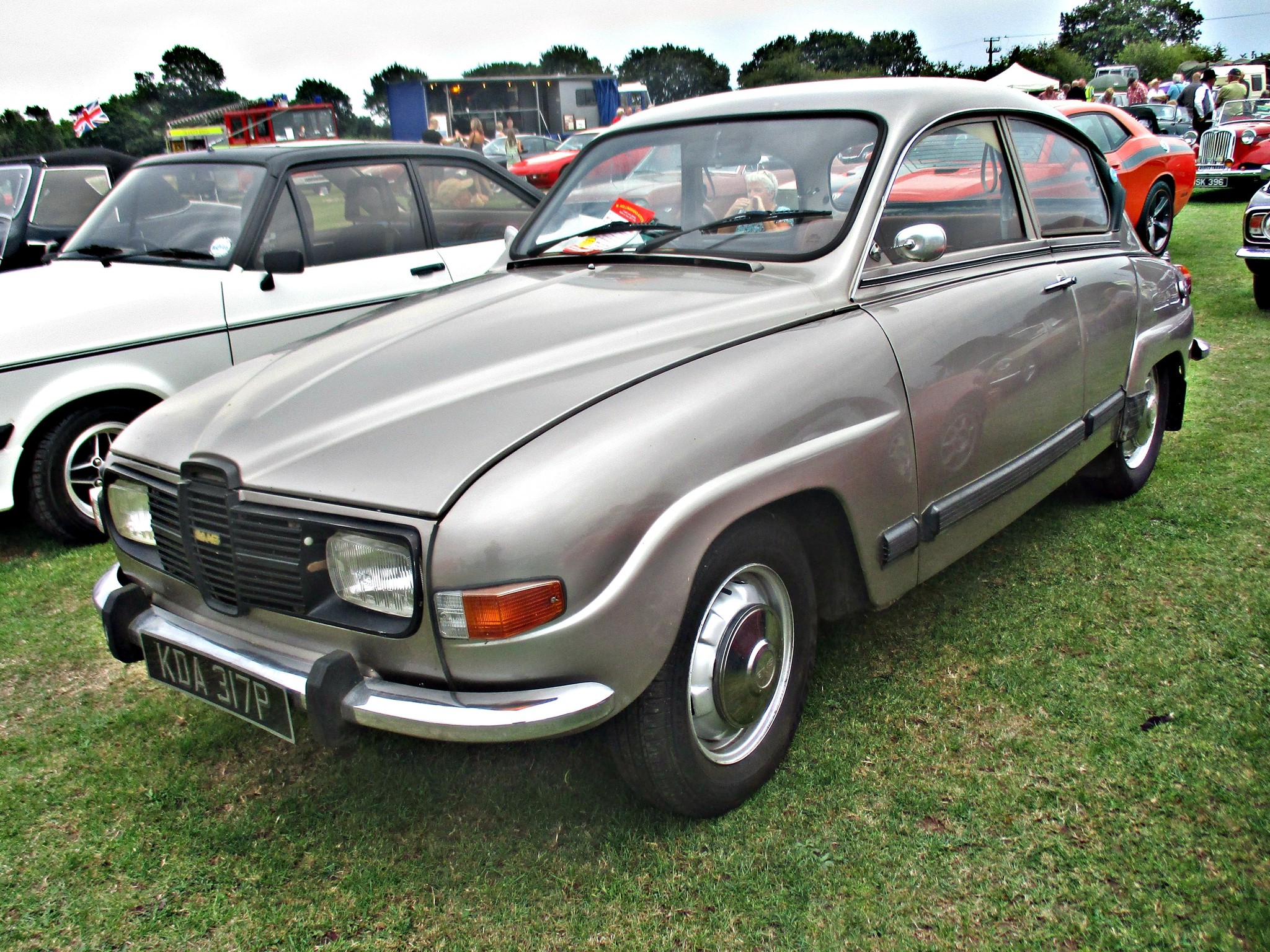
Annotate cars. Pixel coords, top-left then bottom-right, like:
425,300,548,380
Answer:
89,70,1212,821
356,63,1270,259
1233,178,1270,312
1,146,243,276
0,137,599,551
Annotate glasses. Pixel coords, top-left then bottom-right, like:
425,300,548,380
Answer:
750,170,769,177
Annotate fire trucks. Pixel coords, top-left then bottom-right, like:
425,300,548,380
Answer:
162,99,339,203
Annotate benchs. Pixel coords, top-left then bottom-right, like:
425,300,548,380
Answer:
794,212,1092,264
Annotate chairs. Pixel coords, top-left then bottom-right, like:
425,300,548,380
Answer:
240,181,315,255
332,173,400,254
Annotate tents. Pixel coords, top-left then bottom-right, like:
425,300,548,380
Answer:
986,62,1059,95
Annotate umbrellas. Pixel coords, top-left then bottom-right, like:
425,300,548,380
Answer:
1085,73,1128,92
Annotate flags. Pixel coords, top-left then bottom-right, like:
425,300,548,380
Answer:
73,100,110,138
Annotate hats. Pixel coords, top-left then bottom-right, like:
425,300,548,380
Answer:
1150,79,1161,87
435,178,473,204
1226,68,1242,76
1200,70,1218,81
1126,77,1136,88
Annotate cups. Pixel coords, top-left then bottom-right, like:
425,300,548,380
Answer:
743,199,753,212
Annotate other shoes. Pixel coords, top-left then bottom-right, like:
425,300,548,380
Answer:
482,182,502,193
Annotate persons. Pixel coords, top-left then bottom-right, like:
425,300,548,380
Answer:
496,118,519,138
1253,90,1270,114
1036,78,1095,102
505,130,528,182
717,169,794,233
419,119,495,200
436,178,474,207
1097,87,1119,107
1126,68,1250,146
